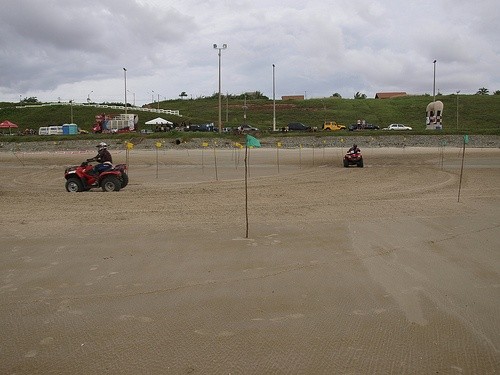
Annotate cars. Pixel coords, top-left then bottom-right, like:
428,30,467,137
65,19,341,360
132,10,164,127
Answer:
236,124,259,131
183,124,230,134
388,123,413,131
348,119,380,130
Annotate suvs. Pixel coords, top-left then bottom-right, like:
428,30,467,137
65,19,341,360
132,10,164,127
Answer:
321,121,347,132
285,122,313,131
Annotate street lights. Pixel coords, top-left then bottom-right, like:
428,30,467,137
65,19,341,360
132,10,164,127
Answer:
271,64,276,132
152,90,160,109
87,90,94,105
433,59,437,102
212,43,228,134
122,67,127,122
127,90,136,106
456,90,461,129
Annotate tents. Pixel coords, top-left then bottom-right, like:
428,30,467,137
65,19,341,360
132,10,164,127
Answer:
0,120,17,134
145,117,173,131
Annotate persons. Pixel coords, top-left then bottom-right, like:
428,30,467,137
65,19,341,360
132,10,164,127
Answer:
347,144,360,154
86,142,113,186
284,124,289,131
307,125,317,132
349,118,366,131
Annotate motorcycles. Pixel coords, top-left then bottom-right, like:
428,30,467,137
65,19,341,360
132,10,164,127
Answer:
64,159,128,192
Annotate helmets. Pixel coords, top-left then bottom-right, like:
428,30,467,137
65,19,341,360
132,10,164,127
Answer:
96,141,107,150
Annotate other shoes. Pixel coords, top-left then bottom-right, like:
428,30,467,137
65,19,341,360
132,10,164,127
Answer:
91,180,99,186
